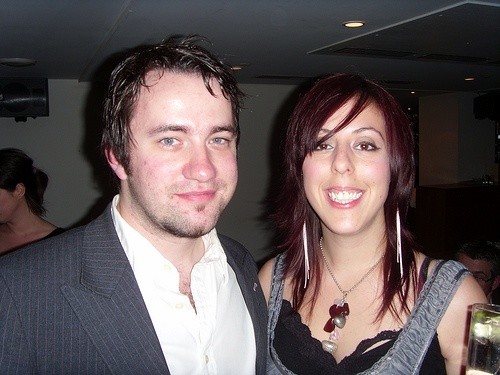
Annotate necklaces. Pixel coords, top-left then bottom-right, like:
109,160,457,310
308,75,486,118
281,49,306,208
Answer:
319,237,386,356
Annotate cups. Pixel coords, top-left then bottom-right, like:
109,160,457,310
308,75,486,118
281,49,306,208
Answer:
466,303,500,375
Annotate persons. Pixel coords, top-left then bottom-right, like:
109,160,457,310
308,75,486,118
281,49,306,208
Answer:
256,69,490,375
0,34,271,375
0,147,65,259
456,240,500,315
